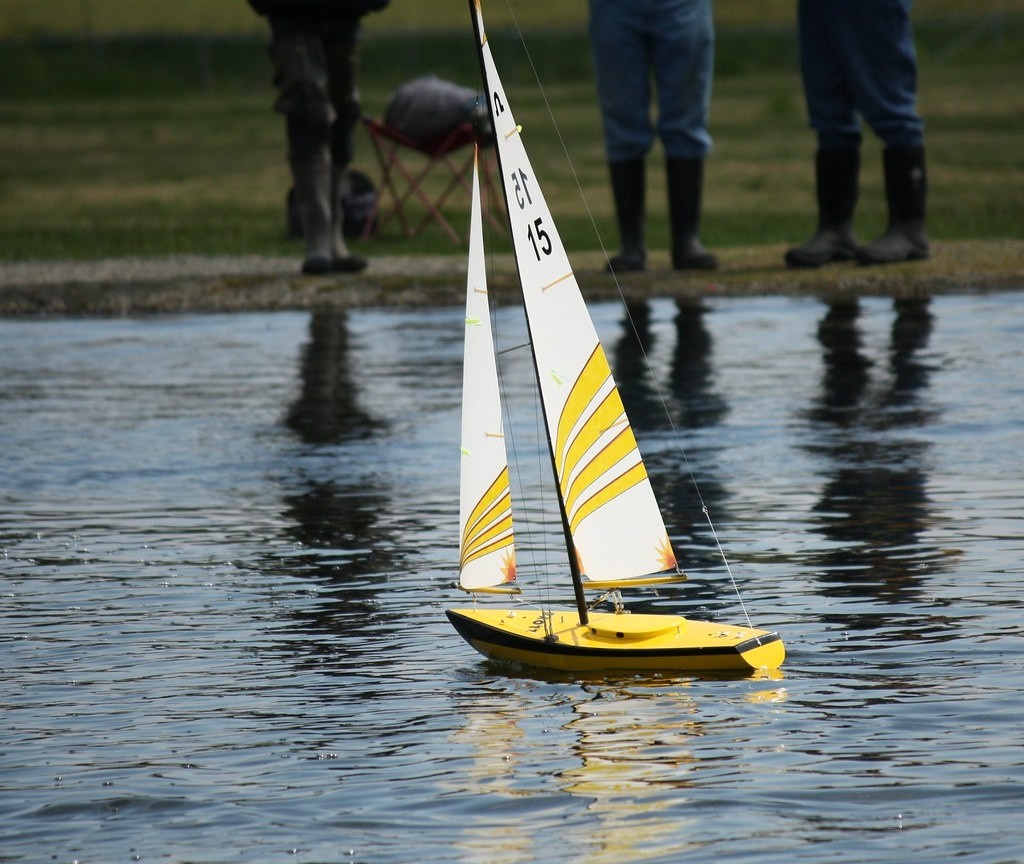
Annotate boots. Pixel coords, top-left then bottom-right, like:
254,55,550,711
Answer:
605,157,648,273
666,156,718,270
857,145,929,264
289,147,332,274
333,164,368,273
784,151,860,266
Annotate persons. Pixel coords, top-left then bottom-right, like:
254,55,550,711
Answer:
784,0,928,269
244,0,392,273
586,0,717,275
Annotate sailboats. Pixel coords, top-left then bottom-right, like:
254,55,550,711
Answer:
438,1,787,673
